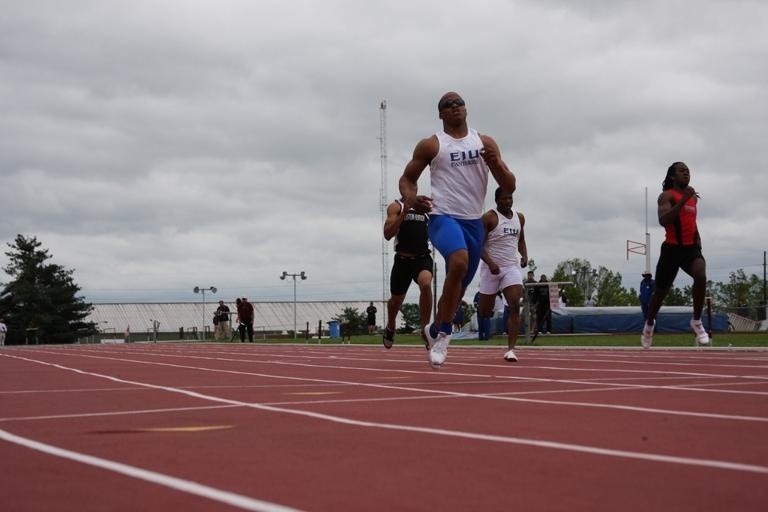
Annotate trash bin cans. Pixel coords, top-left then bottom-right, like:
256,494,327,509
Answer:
327,321,340,339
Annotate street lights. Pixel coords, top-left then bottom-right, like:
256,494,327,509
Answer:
193,285,217,341
280,270,306,338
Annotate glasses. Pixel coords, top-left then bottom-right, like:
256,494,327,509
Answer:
439,99,465,111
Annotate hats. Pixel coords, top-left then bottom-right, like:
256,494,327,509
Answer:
642,271,652,278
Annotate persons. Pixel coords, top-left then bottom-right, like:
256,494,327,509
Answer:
523,271,538,334
0,319,8,346
213,298,254,343
638,271,655,324
478,185,528,362
537,274,551,335
641,163,709,348
382,174,435,349
366,301,377,335
398,93,517,365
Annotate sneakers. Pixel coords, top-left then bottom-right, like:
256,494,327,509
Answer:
383,323,396,348
421,323,452,369
641,318,657,349
504,351,518,361
690,318,709,344
538,331,550,334
